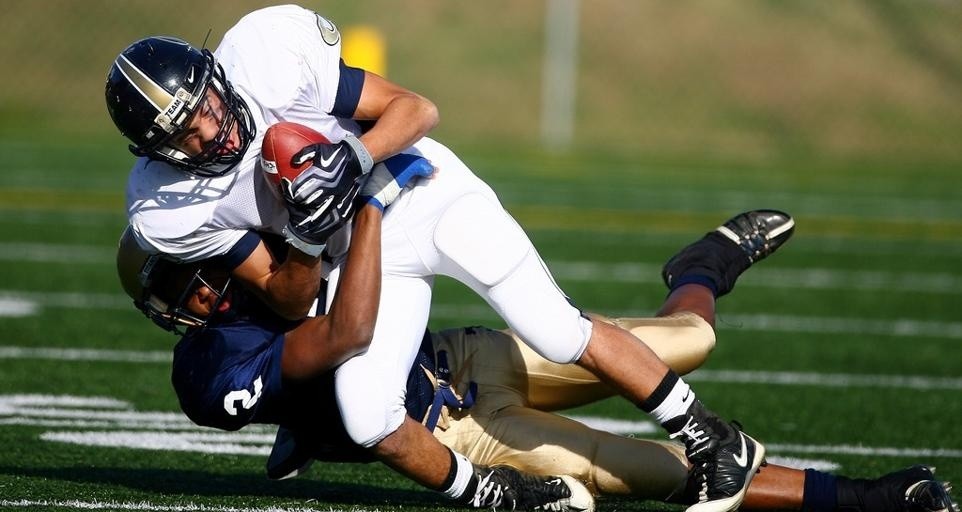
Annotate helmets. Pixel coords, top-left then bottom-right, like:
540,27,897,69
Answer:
105,35,256,177
117,224,231,338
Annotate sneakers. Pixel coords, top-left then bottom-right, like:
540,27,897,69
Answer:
837,465,959,511
661,397,767,512
662,209,795,297
457,463,595,512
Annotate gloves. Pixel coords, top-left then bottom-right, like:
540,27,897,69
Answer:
282,137,434,258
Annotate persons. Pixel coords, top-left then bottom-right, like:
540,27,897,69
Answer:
120,209,961,512
109,7,767,512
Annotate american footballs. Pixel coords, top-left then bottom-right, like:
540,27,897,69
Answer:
261,122,333,209
